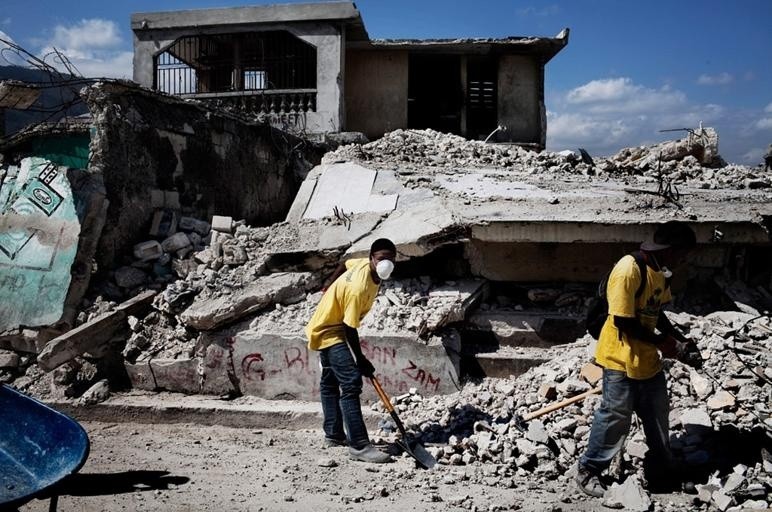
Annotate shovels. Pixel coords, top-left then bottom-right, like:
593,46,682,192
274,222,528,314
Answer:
370,374,436,469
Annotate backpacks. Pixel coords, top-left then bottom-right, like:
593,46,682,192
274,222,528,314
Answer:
585,252,647,339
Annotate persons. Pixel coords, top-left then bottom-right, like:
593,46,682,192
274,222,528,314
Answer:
572,221,701,498
303,237,399,463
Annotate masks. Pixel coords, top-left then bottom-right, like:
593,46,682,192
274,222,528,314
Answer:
376,259,394,280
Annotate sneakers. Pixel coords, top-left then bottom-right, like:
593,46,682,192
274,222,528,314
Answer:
348,447,391,462
682,480,695,492
322,439,347,449
575,460,606,497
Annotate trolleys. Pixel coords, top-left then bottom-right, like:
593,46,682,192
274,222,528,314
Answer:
0,380,91,511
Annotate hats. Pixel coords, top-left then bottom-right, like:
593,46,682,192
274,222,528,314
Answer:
641,220,696,250
